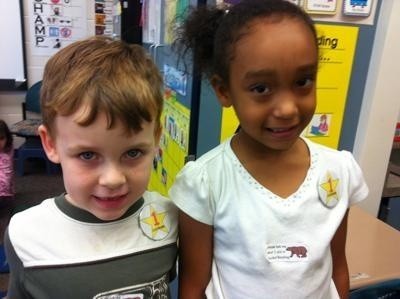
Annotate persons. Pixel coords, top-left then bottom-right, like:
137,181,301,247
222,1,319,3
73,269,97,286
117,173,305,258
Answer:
4,36,179,299
0,119,15,218
168,0,371,299
